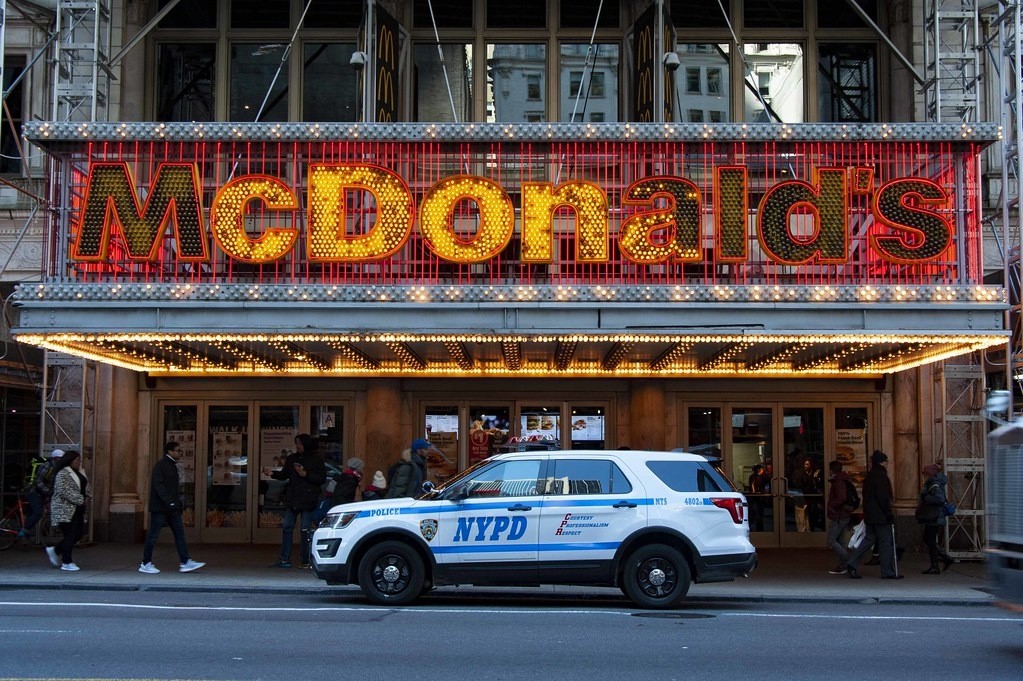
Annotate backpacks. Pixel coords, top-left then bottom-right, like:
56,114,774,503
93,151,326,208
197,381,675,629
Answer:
21,456,54,501
387,462,414,486
844,479,861,511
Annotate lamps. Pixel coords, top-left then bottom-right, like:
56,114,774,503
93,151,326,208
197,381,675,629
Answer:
350,52,369,70
662,52,683,70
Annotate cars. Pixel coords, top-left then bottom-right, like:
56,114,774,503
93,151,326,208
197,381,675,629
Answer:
258,465,289,509
321,462,342,479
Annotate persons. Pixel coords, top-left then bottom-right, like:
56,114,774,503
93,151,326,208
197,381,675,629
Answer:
827,461,850,574
748,464,773,532
17,449,65,545
265,434,326,569
915,464,954,575
361,471,386,501
328,457,364,509
388,439,432,499
798,458,821,532
846,450,904,579
139,442,206,574
45,451,93,571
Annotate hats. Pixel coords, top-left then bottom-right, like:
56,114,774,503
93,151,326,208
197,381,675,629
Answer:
348,457,365,473
872,450,888,463
51,448,66,457
372,470,387,489
924,462,941,475
412,438,432,450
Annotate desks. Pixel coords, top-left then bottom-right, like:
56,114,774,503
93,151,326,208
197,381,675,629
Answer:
745,494,824,531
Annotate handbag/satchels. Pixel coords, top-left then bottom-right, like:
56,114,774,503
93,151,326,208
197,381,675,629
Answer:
914,483,940,523
944,501,955,516
312,496,330,525
848,518,875,550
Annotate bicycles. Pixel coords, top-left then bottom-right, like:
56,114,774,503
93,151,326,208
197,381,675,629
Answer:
0,486,81,549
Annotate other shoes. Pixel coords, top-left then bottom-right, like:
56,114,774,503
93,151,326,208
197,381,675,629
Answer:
893,546,907,567
848,565,862,579
864,556,880,565
827,564,847,574
943,555,954,571
298,560,310,568
46,545,60,566
881,573,904,579
921,563,941,574
61,561,80,571
269,558,292,568
19,529,35,542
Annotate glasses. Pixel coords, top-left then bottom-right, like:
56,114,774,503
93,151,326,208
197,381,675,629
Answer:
173,449,183,454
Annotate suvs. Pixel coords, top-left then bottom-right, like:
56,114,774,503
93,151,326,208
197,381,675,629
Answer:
309,450,762,607
207,457,248,511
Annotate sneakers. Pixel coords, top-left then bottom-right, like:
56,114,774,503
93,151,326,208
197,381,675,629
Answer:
178,558,207,572
139,561,160,573
